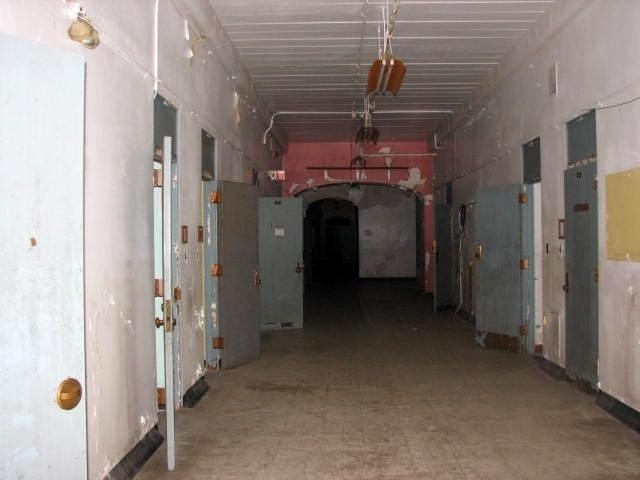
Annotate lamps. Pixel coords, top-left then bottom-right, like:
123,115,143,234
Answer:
356,95,380,145
364,1,406,98
